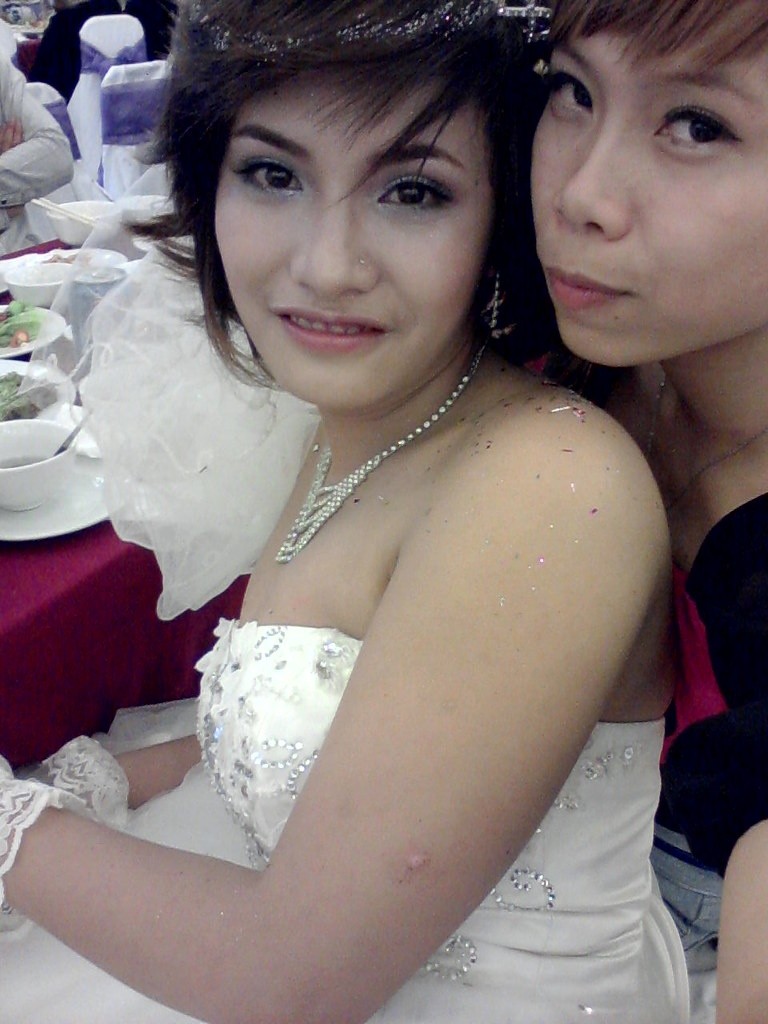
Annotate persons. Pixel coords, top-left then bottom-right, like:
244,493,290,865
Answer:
0,2,767,1024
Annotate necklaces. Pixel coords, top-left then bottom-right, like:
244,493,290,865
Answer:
644,373,768,510
274,345,491,565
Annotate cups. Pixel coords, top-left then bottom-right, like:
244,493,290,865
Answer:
0,419,77,510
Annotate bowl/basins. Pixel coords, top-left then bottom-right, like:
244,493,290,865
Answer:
48,201,123,248
119,195,172,224
5,263,72,307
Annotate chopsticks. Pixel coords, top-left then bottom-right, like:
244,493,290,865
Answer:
31,197,94,226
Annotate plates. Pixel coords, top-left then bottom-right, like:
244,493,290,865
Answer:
0,360,76,420
50,249,128,283
0,454,122,541
21,28,44,35
0,305,67,359
